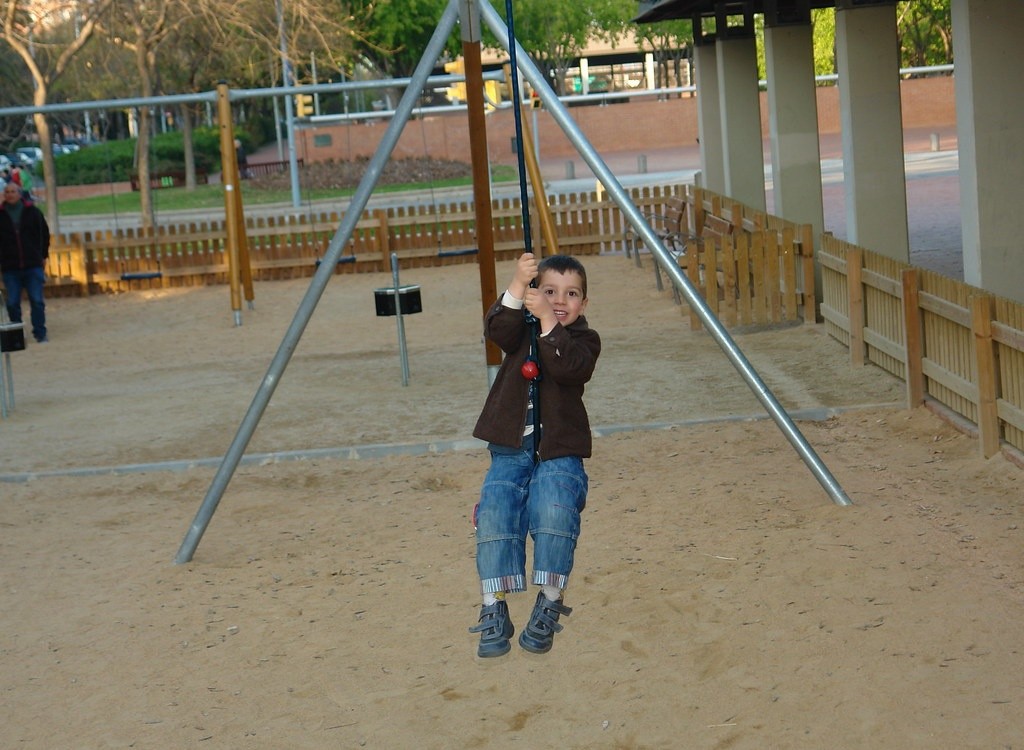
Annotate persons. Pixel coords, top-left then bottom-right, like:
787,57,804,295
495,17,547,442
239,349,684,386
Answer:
0,182,51,343
470,251,602,657
0,162,37,206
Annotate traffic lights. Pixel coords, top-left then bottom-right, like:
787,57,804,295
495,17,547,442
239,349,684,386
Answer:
444,56,465,103
531,85,541,109
294,83,313,118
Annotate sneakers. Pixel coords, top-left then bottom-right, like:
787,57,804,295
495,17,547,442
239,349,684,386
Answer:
469,600,514,658
518,589,572,654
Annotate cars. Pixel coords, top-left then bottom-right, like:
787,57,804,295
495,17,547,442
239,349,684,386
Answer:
0,139,90,176
